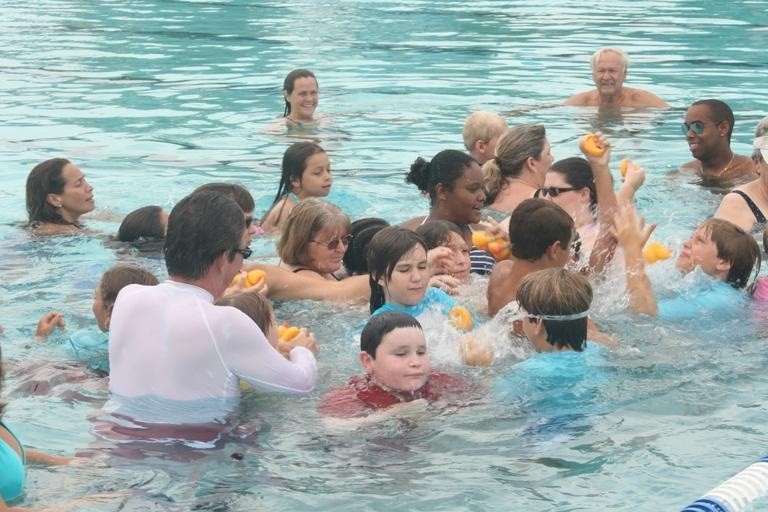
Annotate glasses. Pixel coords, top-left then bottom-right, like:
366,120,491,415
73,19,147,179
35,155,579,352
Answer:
680,122,704,134
232,218,353,259
541,186,576,197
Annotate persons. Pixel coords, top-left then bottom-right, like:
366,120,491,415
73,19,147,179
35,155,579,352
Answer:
260,68,424,156
22,110,658,426
1,408,137,511
554,46,674,138
675,100,768,302
